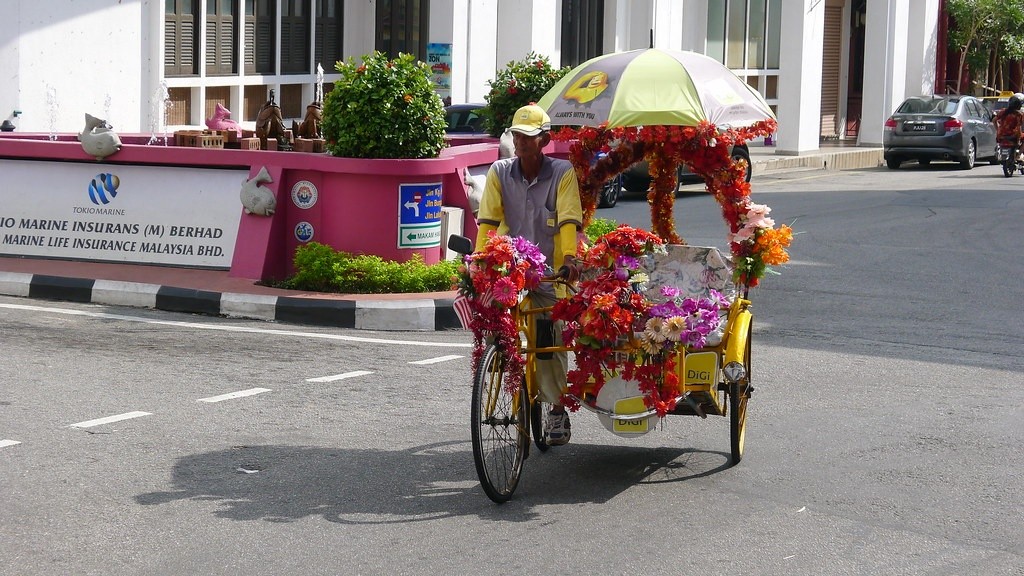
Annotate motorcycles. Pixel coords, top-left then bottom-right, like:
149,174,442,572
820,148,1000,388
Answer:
996,135,1024,178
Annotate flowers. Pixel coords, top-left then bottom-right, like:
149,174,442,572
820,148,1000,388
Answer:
445,117,807,431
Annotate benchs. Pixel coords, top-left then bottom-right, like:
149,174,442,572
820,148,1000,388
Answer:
642,244,735,349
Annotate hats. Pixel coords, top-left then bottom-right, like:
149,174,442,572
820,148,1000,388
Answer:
508,102,551,137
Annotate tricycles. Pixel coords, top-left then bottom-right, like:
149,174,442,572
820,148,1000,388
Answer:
445,234,754,504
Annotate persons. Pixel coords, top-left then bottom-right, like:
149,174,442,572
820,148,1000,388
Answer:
474,105,582,446
992,93,1024,161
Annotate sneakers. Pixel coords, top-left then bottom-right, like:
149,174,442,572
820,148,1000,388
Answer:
487,356,502,372
545,410,571,445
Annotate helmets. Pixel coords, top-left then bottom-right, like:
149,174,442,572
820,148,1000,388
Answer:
1015,93,1024,104
1009,95,1023,109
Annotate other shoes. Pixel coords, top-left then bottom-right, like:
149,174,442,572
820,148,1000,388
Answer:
1016,156,1024,161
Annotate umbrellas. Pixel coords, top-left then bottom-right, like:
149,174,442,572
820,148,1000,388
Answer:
535,29,778,130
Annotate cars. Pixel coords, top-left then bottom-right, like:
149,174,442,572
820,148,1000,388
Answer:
975,92,1014,119
882,93,1001,170
617,134,754,200
441,104,624,209
